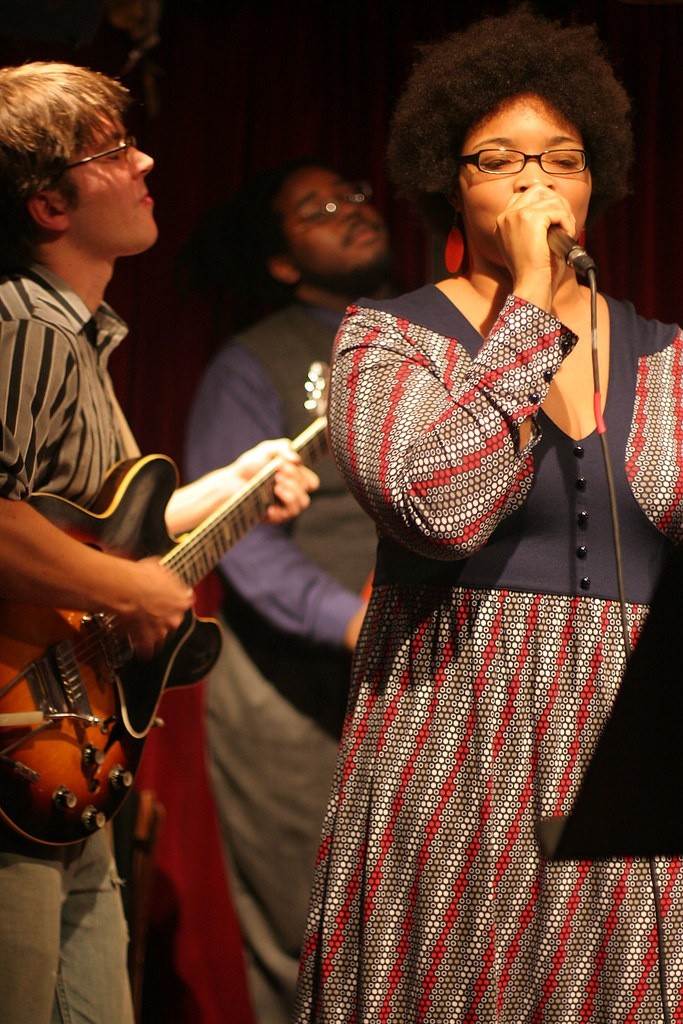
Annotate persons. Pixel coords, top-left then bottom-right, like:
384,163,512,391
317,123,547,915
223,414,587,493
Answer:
0,62,320,1024
182,148,407,1024
285,16,683,1024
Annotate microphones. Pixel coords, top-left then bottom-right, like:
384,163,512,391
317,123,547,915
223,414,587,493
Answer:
547,222,598,278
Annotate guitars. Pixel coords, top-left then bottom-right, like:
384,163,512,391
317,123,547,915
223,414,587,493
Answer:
0,357,329,847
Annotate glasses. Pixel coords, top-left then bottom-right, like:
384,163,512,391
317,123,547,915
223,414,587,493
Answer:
284,189,371,238
49,135,140,187
459,148,591,175
